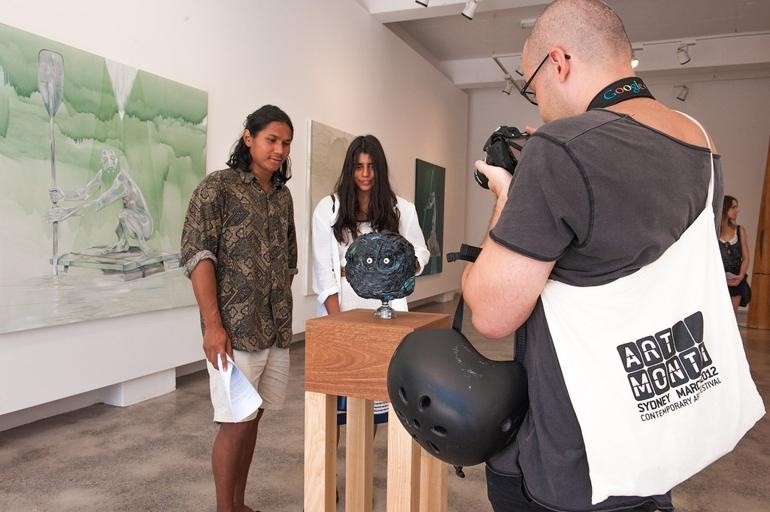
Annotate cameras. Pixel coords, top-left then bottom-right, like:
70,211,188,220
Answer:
474,125,529,188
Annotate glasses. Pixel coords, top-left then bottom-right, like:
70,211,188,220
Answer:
520,52,570,105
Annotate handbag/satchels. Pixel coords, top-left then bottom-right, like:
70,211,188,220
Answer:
739,273,752,307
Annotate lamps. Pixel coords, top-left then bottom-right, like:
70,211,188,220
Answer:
676,44,691,64
415,1,431,8
461,1,478,21
672,84,689,101
515,66,523,75
492,55,522,97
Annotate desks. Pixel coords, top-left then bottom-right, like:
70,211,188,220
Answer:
304,308,452,512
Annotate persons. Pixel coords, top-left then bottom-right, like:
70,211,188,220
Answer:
460,0,724,512
311,134,430,502
50,147,153,255
179,105,298,512
718,196,752,318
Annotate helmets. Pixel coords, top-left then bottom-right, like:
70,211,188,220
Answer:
386,328,531,468
